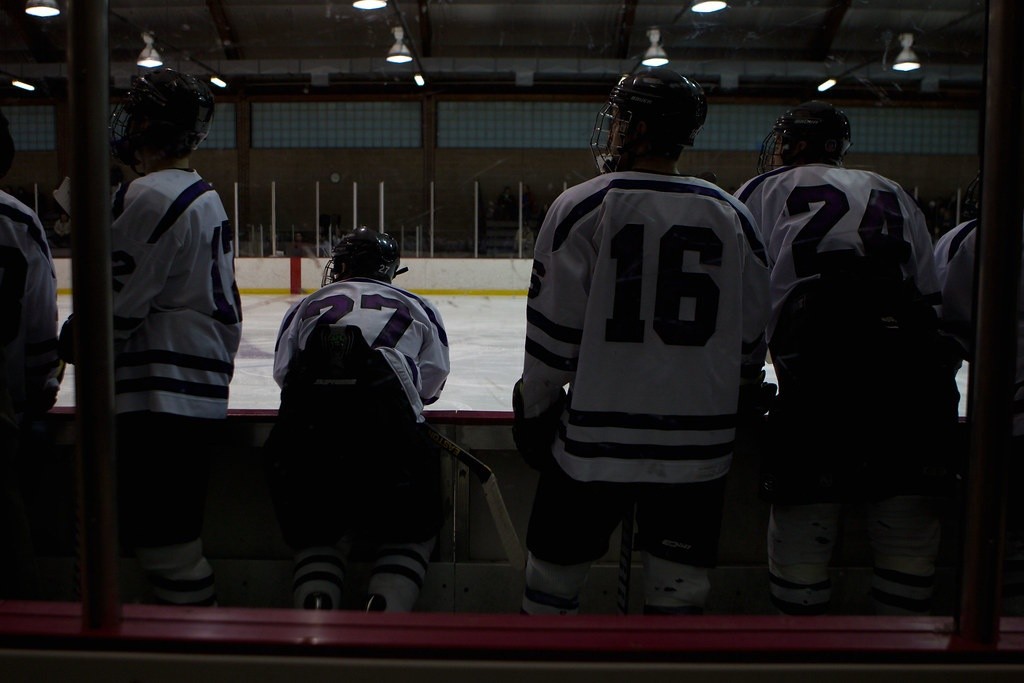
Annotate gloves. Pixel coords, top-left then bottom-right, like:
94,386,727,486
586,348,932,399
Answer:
740,331,782,412
511,377,566,470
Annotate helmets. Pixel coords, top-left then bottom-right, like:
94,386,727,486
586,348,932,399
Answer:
756,100,853,173
108,69,215,166
0,108,15,180
321,230,408,293
589,69,708,173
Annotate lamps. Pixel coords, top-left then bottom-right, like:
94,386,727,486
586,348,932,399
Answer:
385,25,411,63
892,32,921,70
24,0,60,16
641,25,668,66
136,31,162,66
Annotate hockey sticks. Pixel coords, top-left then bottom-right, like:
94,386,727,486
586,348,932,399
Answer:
616,504,633,614
420,422,528,573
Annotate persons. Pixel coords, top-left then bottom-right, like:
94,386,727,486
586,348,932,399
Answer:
62,70,246,611
511,64,776,617
271,225,451,612
734,95,933,615
928,172,1024,608
0,111,72,605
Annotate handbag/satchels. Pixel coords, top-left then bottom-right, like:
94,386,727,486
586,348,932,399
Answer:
496,186,519,219
